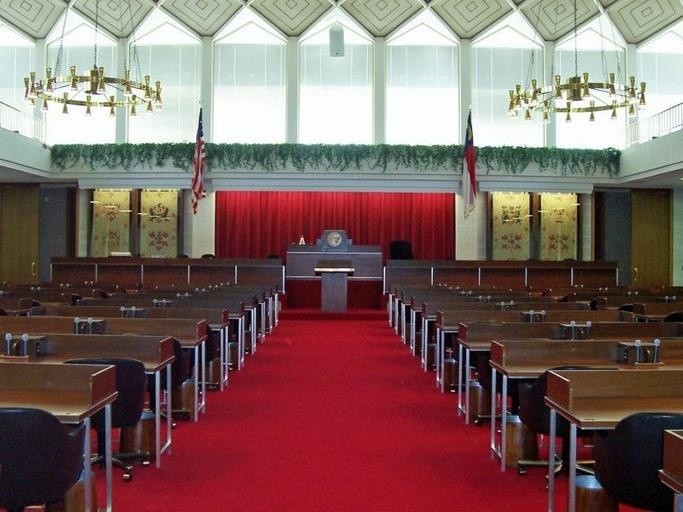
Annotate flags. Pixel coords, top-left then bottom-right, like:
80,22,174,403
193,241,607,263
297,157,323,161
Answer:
463,111,477,219
191,107,206,214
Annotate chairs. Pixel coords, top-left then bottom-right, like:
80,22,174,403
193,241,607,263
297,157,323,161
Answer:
389,240,413,260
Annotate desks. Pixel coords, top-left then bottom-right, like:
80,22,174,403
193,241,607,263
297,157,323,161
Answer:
1,281,275,370
457,320,682,512
1,307,228,512
386,280,683,394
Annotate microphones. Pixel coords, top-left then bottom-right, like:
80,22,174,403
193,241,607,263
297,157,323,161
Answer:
74,317,80,334
6,333,13,356
586,321,591,338
653,339,660,363
120,281,230,318
571,321,575,338
22,334,29,356
88,318,93,334
0,280,94,299
438,283,546,324
635,340,641,363
574,284,677,305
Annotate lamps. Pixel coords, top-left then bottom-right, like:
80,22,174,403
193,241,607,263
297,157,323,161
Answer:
24,0,162,116
508,1,646,122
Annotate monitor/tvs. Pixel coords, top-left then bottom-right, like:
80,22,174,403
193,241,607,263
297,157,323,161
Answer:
111,252,133,257
